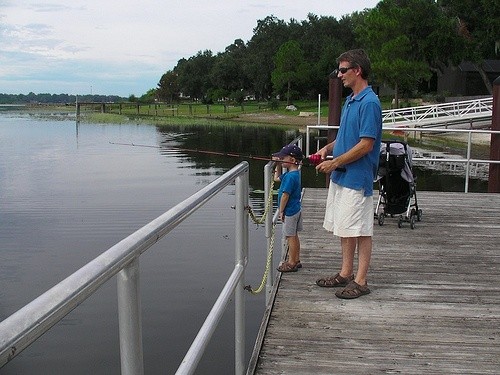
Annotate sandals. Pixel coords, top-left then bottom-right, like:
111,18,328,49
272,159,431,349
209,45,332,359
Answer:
276,264,297,272
335,280,370,299
282,260,303,269
316,274,355,287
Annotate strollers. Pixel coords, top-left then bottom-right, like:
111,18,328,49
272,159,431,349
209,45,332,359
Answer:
373,140,423,229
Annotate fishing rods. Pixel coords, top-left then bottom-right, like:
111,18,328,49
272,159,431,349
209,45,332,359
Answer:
109,142,347,173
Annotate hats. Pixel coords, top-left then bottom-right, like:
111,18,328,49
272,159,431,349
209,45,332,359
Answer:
272,144,303,160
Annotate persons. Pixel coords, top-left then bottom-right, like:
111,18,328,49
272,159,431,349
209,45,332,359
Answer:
313,48,384,299
272,145,303,272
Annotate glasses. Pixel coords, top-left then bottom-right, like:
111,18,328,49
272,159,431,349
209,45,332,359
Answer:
338,67,354,73
